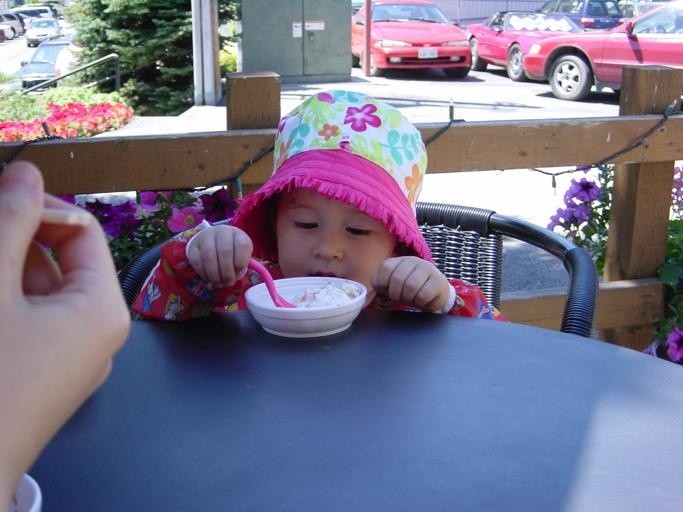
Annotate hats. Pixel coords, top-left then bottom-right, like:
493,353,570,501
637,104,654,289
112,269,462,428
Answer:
227,91,435,265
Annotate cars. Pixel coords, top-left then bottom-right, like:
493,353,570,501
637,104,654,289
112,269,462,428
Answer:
0,6,79,87
352,1,472,80
468,0,682,101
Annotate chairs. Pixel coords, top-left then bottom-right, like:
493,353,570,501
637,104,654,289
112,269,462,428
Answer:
120,201,598,339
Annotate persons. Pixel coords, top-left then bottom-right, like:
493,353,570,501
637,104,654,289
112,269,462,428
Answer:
128,89,507,324
0,158,132,511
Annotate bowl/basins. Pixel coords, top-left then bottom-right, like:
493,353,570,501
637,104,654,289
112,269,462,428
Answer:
245,275,367,340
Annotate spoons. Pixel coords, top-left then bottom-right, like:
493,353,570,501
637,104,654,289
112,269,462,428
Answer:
246,258,295,307
